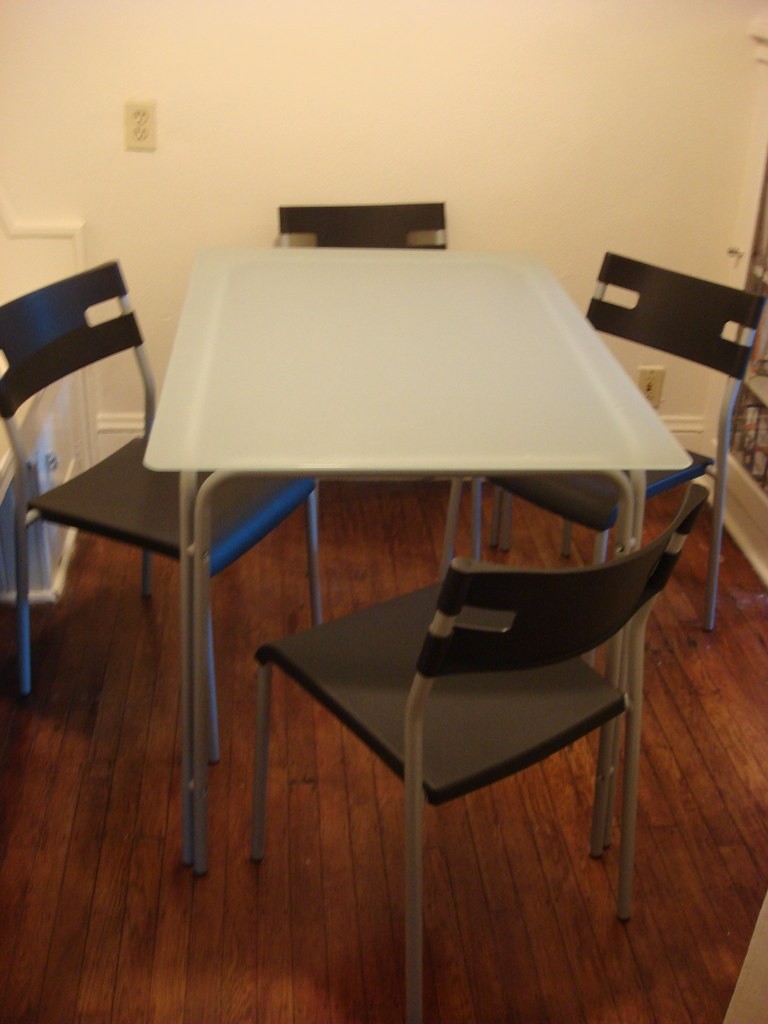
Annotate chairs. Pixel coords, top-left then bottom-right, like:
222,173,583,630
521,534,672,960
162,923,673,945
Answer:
1,260,324,763
276,202,448,249
250,483,711,1024
489,252,767,631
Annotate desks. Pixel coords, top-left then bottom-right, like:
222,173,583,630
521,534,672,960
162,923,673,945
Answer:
144,248,694,877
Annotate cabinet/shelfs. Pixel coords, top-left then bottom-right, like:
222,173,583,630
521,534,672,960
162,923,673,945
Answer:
699,146,768,586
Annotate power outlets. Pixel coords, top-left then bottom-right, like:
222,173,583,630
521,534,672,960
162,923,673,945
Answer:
637,366,665,408
123,98,156,152
